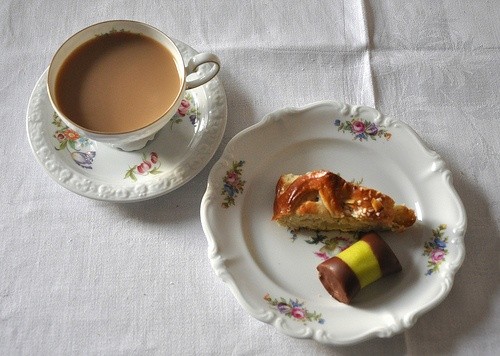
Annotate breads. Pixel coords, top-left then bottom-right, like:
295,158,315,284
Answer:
271,170,416,232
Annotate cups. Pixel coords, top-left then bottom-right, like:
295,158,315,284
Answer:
46,19,221,153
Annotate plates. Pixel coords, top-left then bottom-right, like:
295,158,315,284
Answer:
25,36,227,205
200,100,469,349
